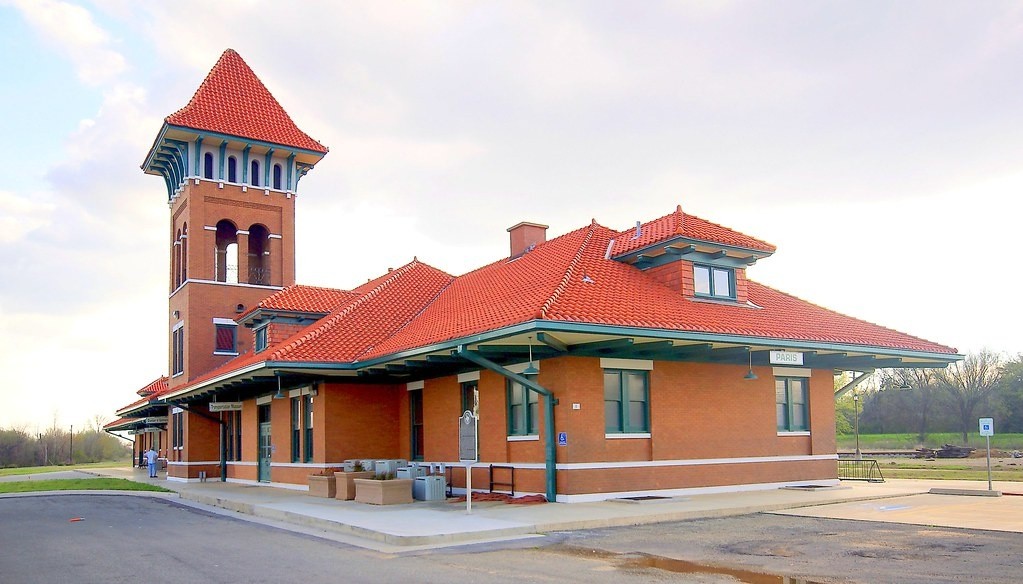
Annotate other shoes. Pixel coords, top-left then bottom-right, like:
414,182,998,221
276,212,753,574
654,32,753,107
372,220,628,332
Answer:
150,476,158,478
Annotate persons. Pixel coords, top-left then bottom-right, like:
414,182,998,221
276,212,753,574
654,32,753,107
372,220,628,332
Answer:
144,447,158,478
